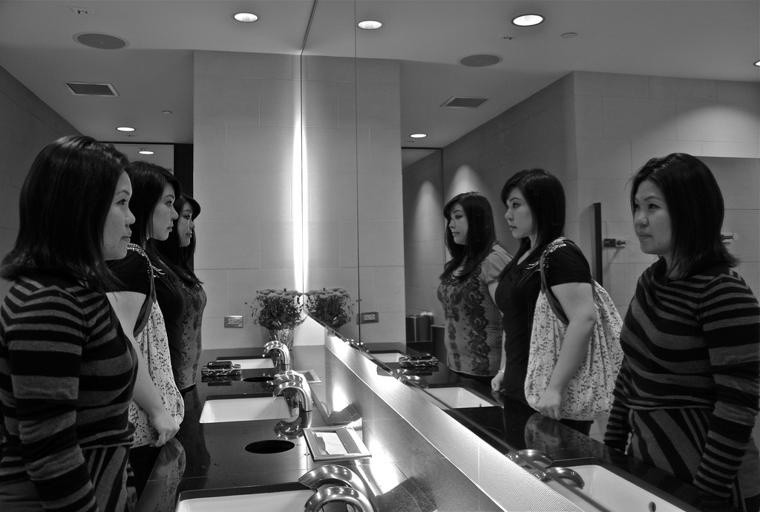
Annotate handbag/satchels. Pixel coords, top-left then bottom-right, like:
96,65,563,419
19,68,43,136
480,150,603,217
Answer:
127,242,184,450
524,236,624,422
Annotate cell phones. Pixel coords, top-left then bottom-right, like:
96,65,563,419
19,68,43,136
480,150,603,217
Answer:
408,351,431,360
208,360,232,369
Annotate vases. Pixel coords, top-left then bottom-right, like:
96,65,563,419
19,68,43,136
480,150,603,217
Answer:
270,327,294,351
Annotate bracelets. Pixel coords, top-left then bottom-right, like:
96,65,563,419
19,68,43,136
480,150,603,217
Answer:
498,368,505,374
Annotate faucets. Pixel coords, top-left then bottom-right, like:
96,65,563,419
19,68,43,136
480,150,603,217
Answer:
261,340,290,365
305,484,373,512
506,449,554,466
390,368,424,385
298,464,368,499
266,372,313,413
275,410,312,441
539,468,584,484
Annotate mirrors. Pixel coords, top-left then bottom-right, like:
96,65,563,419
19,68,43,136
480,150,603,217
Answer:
302,0,760,512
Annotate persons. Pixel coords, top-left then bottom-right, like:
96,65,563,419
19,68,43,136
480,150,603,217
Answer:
0,136,139,512
601,153,760,512
90,161,186,449
148,193,208,398
494,168,596,437
437,192,513,393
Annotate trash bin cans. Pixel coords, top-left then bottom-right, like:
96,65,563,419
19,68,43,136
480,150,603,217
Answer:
407,314,434,344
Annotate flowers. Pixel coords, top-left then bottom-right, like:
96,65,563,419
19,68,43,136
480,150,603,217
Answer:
304,288,362,332
245,289,309,330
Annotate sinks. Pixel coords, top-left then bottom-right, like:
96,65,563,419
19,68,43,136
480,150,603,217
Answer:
173,481,324,512
552,457,698,512
199,394,291,424
366,349,410,364
216,356,275,370
421,384,502,409
